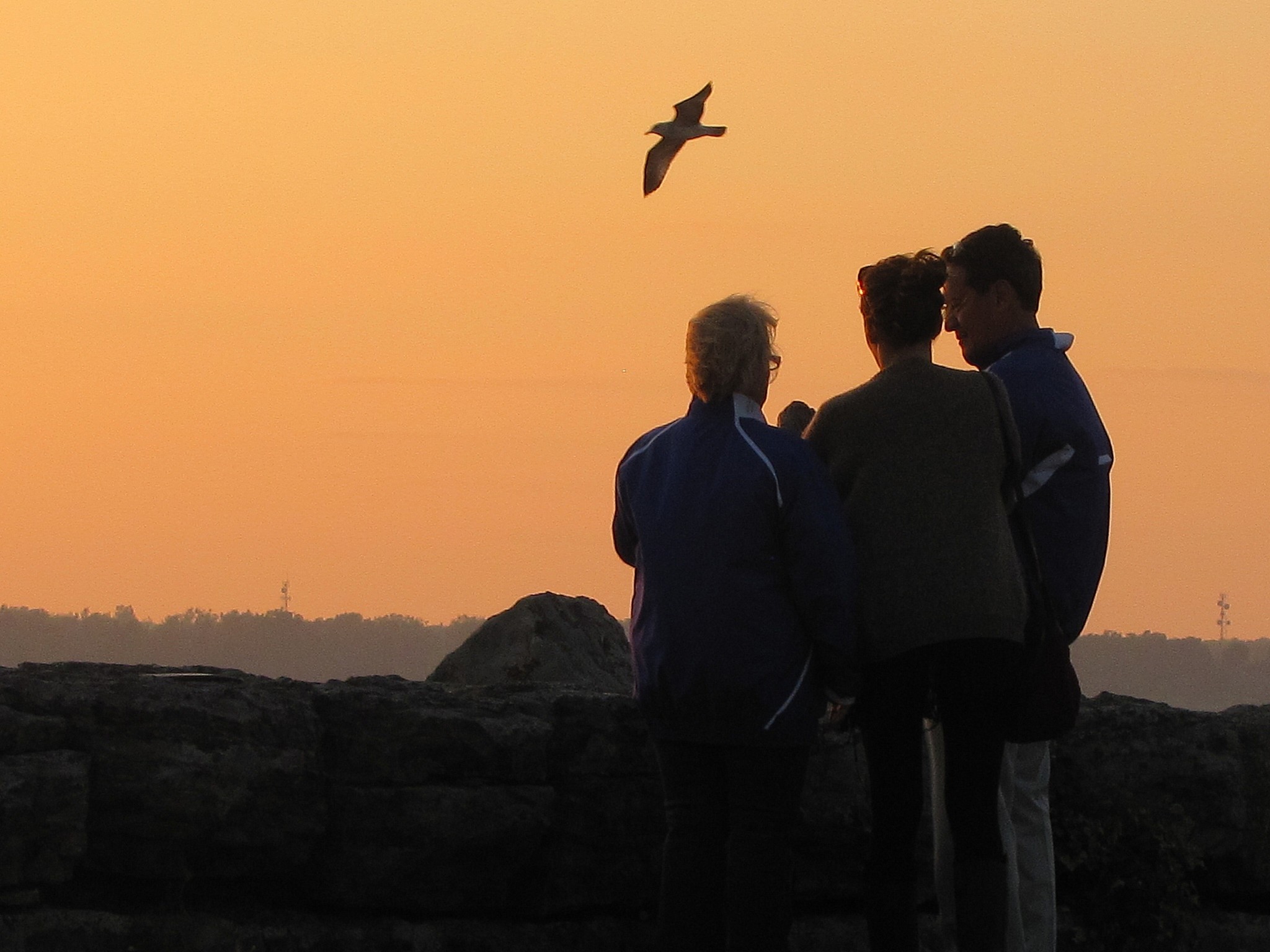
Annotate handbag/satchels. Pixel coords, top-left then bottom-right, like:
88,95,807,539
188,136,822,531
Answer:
987,502,1081,744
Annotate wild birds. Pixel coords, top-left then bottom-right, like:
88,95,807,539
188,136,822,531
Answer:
640,79,730,198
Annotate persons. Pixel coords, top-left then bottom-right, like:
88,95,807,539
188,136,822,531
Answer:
612,225,1114,952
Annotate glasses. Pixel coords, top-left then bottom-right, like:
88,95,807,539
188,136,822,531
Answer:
857,266,876,308
760,352,781,371
